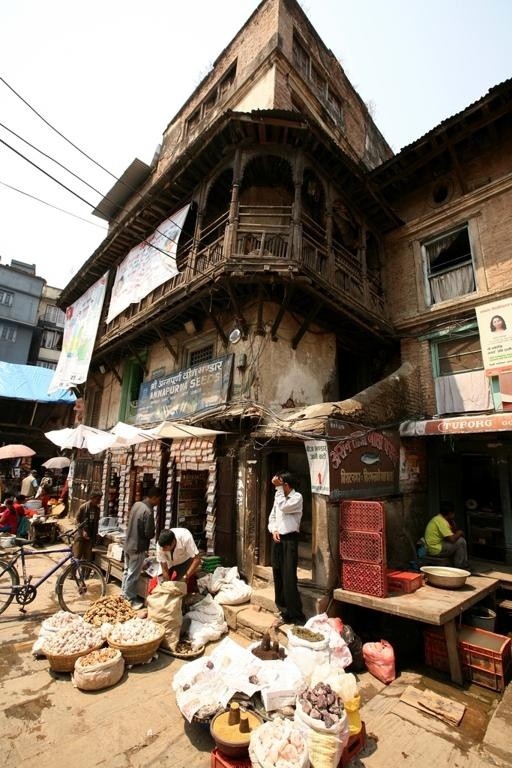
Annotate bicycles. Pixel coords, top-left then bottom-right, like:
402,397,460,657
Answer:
0,514,106,616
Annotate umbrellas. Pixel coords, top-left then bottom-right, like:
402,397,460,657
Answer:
0,444,36,460
41,456,71,470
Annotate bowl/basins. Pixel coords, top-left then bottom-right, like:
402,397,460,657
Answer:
419,565,472,590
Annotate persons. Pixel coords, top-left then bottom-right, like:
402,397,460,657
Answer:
69,489,104,580
0,468,66,539
265,470,307,626
119,486,164,607
423,502,472,572
155,527,202,597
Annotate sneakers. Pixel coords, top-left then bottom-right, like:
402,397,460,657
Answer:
131,602,144,610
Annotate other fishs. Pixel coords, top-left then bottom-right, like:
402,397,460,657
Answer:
360,452,381,466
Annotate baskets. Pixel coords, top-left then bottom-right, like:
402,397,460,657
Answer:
42,634,108,673
106,618,164,666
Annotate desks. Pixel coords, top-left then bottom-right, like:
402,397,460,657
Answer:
332,566,502,688
91,543,149,601
